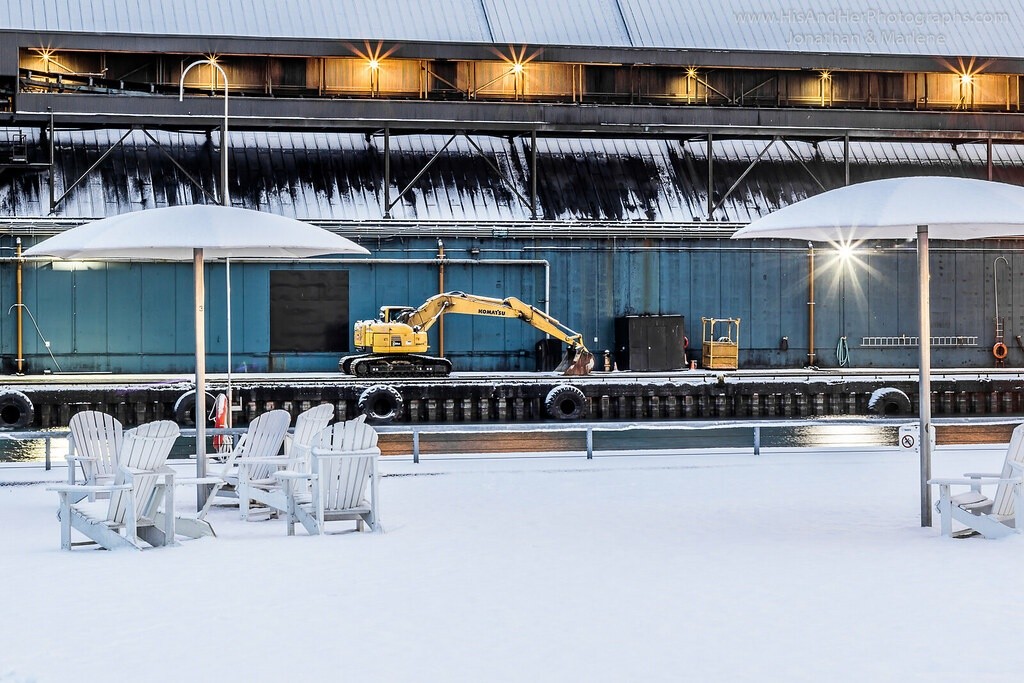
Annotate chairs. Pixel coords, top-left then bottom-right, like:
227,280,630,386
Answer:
46,403,382,552
927,424,1023,540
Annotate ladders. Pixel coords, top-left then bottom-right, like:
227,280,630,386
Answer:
995,317,1005,368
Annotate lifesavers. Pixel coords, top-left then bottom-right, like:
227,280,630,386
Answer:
358,384,404,423
545,385,589,420
993,343,1007,359
0,389,34,429
683,335,689,348
868,387,912,415
174,390,216,427
213,394,227,450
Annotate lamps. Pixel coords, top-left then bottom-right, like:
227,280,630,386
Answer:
472,248,481,254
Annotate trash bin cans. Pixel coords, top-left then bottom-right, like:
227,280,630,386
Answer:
535,338,562,371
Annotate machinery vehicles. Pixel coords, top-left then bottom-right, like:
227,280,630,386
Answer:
335,293,596,378
